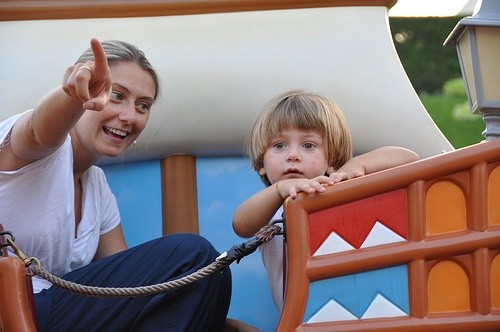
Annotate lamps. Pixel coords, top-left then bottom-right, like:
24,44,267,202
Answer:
443,0,500,141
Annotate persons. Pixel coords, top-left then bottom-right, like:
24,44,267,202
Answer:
232,91,420,320
0,38,232,332
